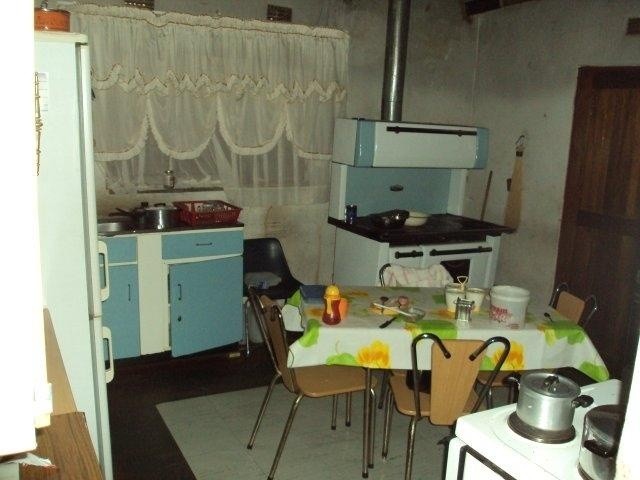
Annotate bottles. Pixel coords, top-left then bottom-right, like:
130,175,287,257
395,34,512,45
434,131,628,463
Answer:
323,285,341,325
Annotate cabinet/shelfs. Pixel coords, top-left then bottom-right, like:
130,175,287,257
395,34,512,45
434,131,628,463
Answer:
96,237,143,359
160,232,246,357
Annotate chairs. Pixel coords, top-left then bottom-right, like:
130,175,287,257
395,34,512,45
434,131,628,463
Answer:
251,297,377,479
374,257,464,406
476,284,599,421
241,232,307,356
382,334,511,480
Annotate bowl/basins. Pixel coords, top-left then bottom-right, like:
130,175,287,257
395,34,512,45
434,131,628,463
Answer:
406,211,431,227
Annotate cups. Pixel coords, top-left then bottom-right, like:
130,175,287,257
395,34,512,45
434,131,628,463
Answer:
453,300,475,327
466,286,484,312
446,290,466,315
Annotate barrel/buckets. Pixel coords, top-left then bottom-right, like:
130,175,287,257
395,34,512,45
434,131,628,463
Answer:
488,284,530,330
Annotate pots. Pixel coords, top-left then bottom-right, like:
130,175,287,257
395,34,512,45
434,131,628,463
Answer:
133,201,150,229
146,202,171,230
504,370,603,431
575,405,625,480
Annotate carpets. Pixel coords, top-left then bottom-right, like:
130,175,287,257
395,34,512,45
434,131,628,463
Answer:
155,367,518,480
